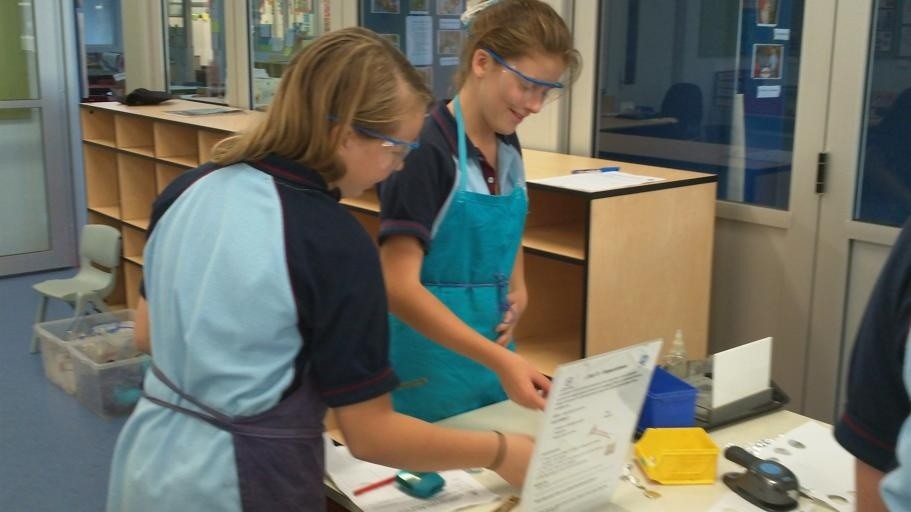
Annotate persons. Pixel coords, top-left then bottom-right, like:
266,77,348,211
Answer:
831,214,911,512
373,0,584,423
103,24,537,511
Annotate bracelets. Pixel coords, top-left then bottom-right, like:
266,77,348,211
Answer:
486,430,507,471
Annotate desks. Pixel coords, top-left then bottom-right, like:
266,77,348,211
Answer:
322,359,856,512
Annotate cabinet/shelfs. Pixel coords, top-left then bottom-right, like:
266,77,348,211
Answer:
597,113,793,211
79,94,717,381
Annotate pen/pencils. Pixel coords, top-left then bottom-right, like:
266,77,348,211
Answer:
352,475,397,497
571,167,619,174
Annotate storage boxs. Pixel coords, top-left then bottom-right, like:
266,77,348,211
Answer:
33,309,154,420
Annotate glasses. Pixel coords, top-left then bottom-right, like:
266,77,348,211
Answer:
486,47,566,107
320,110,420,168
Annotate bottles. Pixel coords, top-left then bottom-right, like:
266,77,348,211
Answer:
662,326,691,383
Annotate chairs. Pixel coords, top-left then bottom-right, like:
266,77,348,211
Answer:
30,224,122,354
